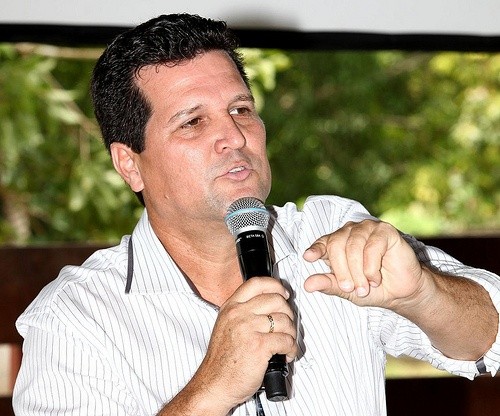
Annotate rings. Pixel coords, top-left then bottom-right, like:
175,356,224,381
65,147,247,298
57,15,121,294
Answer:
265,314,275,333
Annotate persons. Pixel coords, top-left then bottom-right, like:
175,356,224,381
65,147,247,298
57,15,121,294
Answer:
11,12,500,416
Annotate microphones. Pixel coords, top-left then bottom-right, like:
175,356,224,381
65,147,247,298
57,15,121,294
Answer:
224,197,288,401
